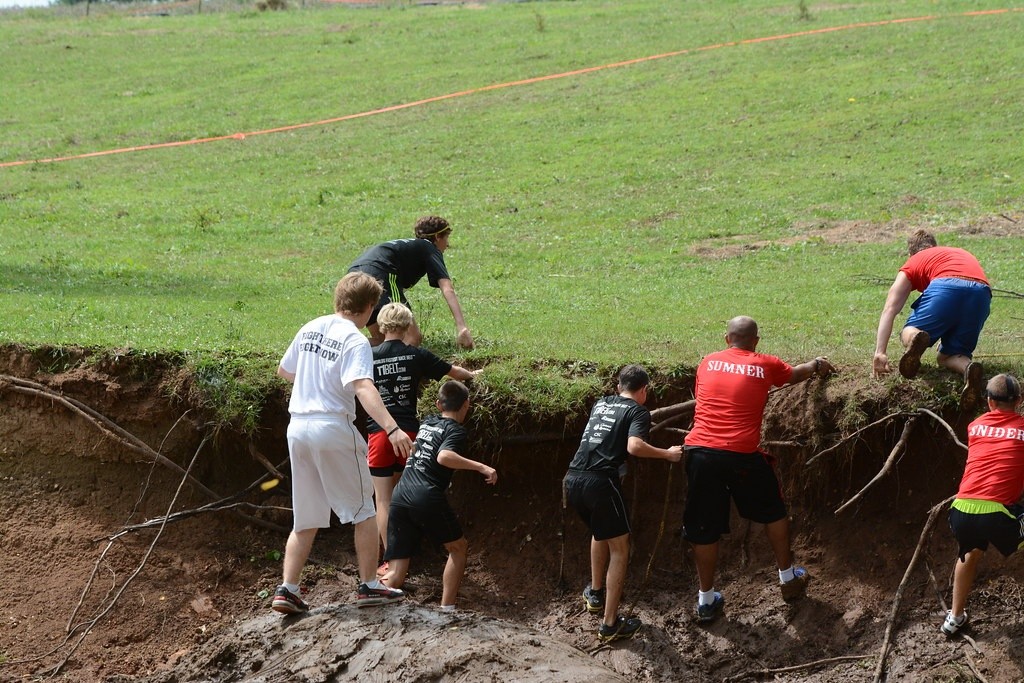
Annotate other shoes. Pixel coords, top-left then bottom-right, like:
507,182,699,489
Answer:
899,329,929,379
962,363,983,415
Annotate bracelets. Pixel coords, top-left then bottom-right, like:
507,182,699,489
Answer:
386,426,400,438
815,359,821,372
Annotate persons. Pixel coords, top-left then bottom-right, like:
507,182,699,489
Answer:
348,216,475,352
941,374,1024,635
366,304,483,575
381,381,497,611
872,229,993,412
272,272,415,614
565,364,682,639
684,315,835,618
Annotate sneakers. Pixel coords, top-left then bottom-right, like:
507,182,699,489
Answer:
377,561,390,576
940,607,968,636
272,584,308,615
597,615,641,642
582,582,604,612
699,592,722,621
781,568,807,600
357,577,406,608
